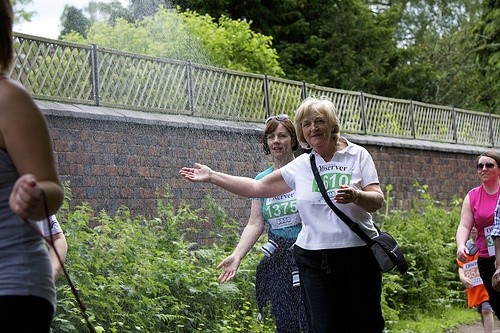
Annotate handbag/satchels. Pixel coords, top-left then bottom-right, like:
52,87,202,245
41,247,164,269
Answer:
365,232,408,276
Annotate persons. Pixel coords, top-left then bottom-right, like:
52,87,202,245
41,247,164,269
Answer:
456,151,500,333
215,114,308,333
0,0,69,333
179,96,386,333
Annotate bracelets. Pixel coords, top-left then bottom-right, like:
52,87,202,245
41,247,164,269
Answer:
354,189,359,203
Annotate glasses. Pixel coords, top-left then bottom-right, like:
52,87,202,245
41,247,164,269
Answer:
265,115,289,129
477,163,498,169
